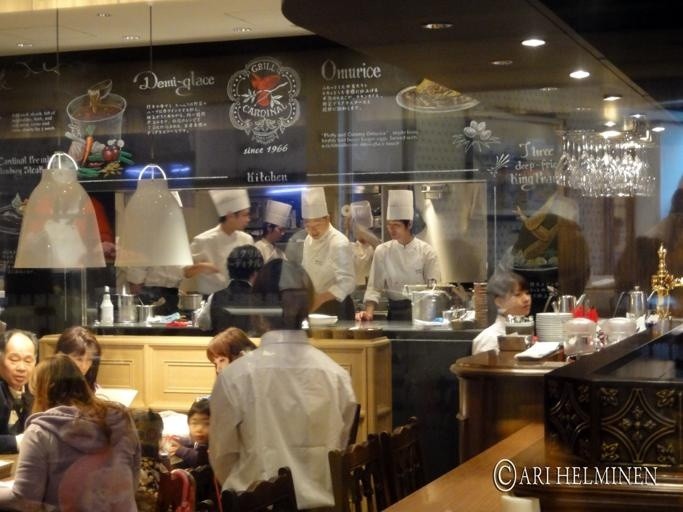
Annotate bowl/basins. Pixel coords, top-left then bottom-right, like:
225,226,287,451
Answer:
113,292,203,322
404,284,455,323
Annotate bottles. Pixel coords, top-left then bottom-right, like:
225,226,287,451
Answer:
98,285,113,324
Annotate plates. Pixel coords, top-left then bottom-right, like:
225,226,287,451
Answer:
394,85,481,112
307,313,338,326
534,311,573,343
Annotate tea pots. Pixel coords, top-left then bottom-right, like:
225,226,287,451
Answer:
550,291,586,313
609,285,657,318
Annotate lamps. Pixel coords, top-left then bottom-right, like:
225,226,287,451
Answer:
13,9,107,270
112,2,194,267
649,121,665,133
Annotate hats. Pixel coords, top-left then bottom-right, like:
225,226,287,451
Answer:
386,189,414,221
208,189,250,218
264,200,292,228
351,200,373,228
301,187,329,219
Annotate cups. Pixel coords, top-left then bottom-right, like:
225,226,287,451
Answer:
66,94,126,145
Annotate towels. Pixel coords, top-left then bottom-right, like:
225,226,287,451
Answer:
514,339,559,361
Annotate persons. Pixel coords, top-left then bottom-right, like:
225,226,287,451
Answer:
54,326,100,392
204,326,257,373
169,398,219,499
471,270,534,355
205,258,358,510
129,408,170,512
0,328,39,454
94,187,442,335
0,356,142,511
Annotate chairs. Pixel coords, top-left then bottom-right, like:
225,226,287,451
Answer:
329,433,387,512
156,468,196,511
221,465,298,512
380,416,426,503
